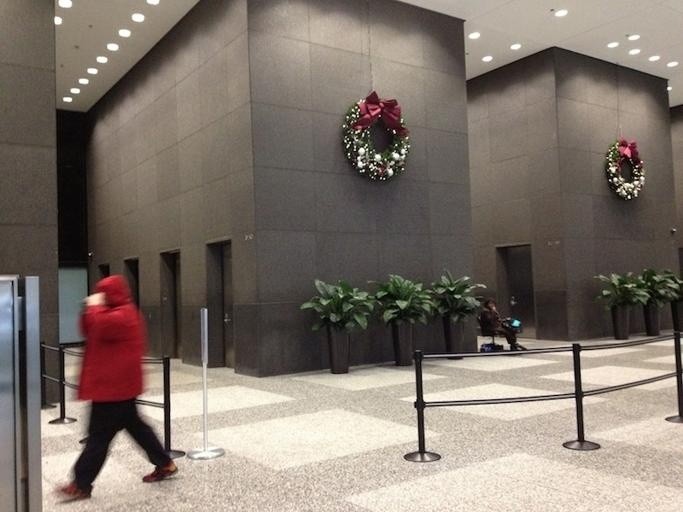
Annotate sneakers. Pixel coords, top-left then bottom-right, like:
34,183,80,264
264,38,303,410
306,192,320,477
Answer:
53,481,94,504
142,460,180,484
510,343,527,351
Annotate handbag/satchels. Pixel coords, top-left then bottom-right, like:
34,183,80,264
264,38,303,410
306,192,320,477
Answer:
502,315,523,334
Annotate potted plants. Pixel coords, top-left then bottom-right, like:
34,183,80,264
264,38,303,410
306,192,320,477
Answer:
299,276,383,375
366,272,439,367
590,269,653,341
634,267,683,337
424,268,489,360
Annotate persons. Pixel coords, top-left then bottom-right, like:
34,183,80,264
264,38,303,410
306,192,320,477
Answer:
57,274,178,498
480,298,519,352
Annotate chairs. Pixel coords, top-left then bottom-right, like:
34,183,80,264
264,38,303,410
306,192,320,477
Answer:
474,308,504,353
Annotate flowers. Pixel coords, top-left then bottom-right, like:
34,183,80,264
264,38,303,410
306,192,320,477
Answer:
340,93,414,186
603,138,649,202
